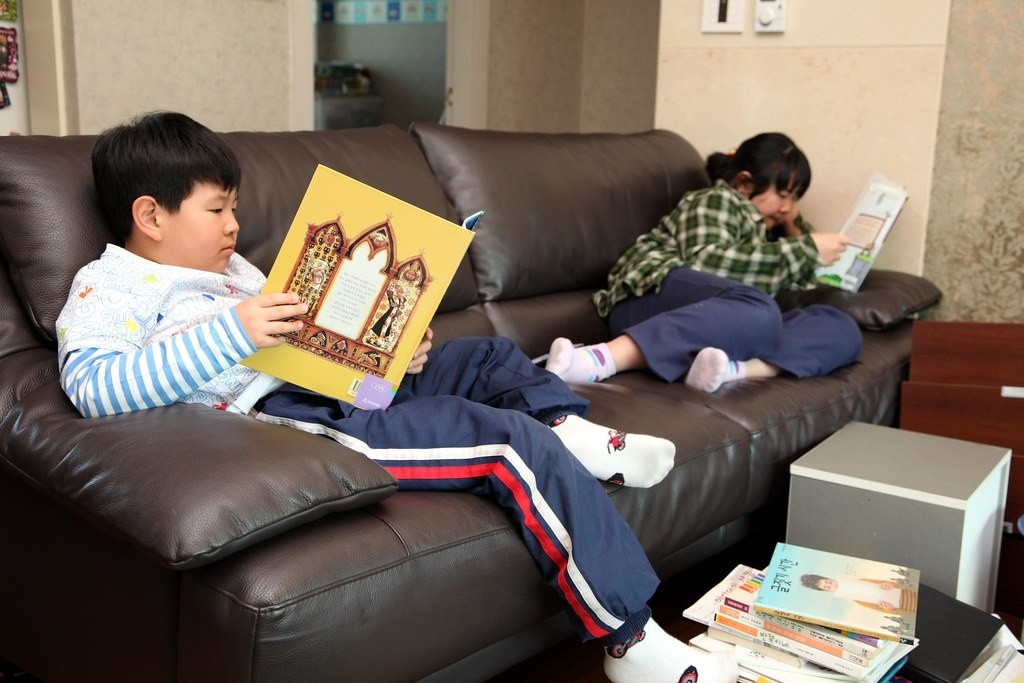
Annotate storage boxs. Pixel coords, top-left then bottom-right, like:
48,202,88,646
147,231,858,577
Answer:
315,98,382,131
786,419,1013,616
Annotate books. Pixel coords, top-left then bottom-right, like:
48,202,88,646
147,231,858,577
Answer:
751,541,921,643
812,179,909,294
236,163,488,411
682,563,920,683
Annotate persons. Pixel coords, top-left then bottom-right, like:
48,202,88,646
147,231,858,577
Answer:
53,108,745,683
544,132,865,391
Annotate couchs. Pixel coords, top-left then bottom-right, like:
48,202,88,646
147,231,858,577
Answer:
0,130,943,683
